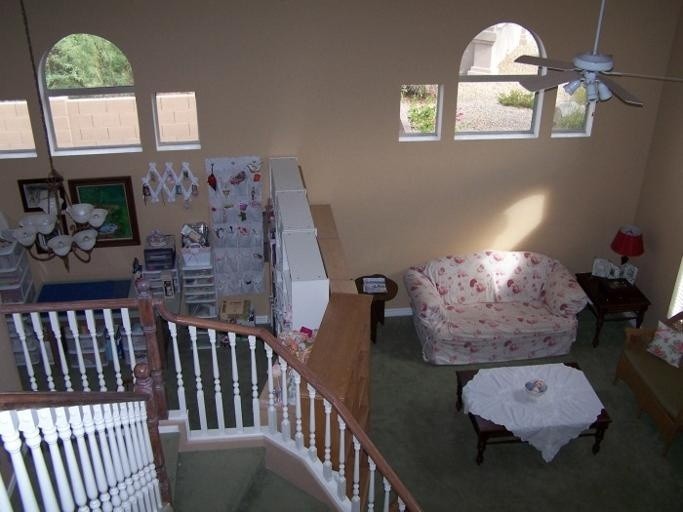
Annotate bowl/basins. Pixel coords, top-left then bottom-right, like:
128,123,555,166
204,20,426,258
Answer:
523,378,549,400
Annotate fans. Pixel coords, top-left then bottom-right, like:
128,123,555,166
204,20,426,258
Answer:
514,0,683,106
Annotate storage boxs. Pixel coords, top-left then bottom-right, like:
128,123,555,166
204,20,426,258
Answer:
0,237,40,367
64,322,149,368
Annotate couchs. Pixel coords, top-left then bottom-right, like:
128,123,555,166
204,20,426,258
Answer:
611,311,683,457
402,251,587,366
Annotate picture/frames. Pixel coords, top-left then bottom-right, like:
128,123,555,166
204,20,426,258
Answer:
16,178,68,212
68,174,141,248
35,214,69,254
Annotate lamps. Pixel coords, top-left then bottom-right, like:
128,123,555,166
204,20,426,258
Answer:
9,0,108,269
563,71,613,102
610,224,644,287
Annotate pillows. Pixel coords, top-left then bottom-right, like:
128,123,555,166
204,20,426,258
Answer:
649,320,683,368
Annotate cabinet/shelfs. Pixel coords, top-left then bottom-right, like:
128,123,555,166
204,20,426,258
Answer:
141,258,181,294
303,203,373,512
267,156,329,357
177,249,221,350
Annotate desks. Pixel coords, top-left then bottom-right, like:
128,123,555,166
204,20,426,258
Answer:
355,274,398,343
26,278,182,376
575,271,651,347
